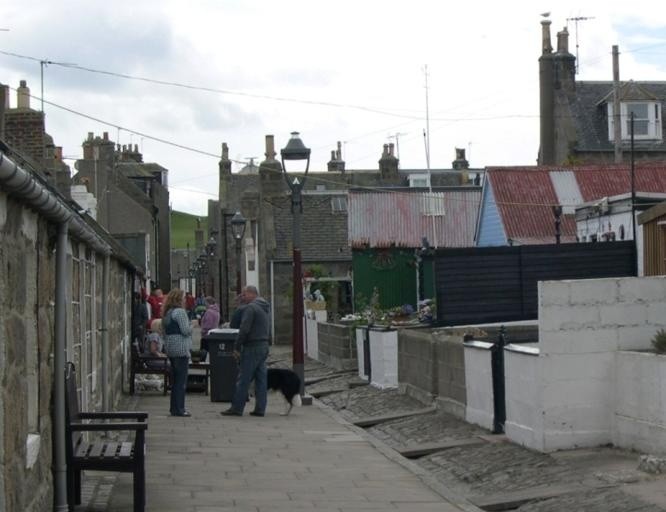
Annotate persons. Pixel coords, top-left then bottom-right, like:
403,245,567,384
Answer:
220,285,270,419
199,296,220,362
183,293,195,320
228,285,248,329
141,277,164,320
145,318,174,390
160,287,198,416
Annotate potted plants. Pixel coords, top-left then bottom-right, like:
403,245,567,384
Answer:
191,350,201,364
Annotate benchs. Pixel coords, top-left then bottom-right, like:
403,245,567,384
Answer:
49,361,149,512
129,338,167,396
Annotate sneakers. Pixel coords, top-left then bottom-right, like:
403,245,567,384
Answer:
220,405,268,417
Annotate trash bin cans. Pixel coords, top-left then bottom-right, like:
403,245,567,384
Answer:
208,329,240,401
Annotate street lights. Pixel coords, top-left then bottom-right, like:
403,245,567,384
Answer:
281,132,312,405
188,236,216,299
231,210,246,295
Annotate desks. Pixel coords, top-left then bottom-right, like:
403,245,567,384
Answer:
188,361,210,397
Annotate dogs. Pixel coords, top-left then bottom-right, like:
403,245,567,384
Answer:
232,350,302,416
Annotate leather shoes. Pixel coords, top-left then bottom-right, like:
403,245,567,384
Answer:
171,410,192,417
